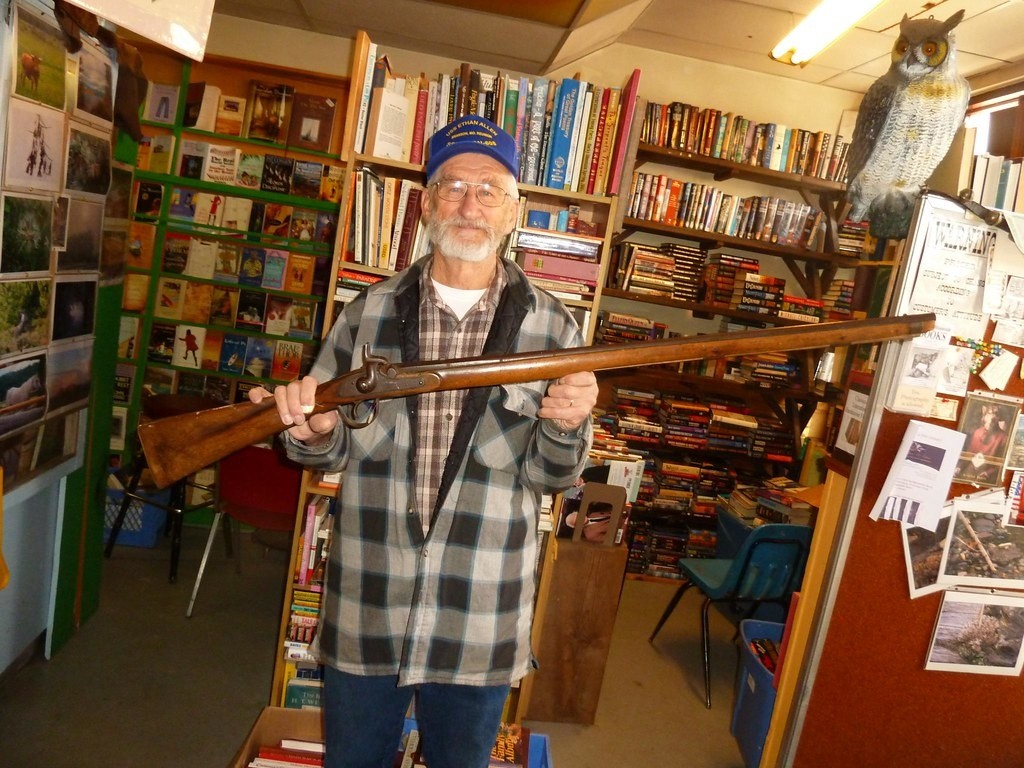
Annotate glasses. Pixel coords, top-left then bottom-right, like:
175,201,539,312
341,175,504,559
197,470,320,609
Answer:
427,178,519,207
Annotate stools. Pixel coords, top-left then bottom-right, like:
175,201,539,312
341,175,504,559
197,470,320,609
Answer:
104,393,238,583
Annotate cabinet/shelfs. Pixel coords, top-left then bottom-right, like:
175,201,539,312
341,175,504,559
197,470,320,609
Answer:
558,95,889,586
270,465,564,724
319,29,642,349
520,537,627,727
111,36,349,532
761,190,1024,768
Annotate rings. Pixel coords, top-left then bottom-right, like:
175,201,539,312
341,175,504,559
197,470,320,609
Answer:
569,399,573,408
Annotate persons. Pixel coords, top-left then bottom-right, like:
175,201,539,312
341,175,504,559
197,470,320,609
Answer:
248,114,598,767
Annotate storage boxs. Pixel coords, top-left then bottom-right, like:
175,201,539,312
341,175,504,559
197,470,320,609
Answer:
104,468,171,548
730,619,786,768
228,706,326,768
395,718,553,768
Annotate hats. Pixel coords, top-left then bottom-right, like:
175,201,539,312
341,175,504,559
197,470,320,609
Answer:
427,115,520,181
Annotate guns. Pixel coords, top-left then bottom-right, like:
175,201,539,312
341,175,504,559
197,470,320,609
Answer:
136,312,941,491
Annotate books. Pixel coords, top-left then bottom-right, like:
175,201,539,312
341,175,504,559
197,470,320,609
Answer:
109,60,1024,768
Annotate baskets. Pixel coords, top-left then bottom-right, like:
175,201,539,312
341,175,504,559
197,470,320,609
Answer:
101,465,170,547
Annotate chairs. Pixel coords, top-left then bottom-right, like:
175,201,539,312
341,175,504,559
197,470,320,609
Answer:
648,523,813,709
186,445,307,620
